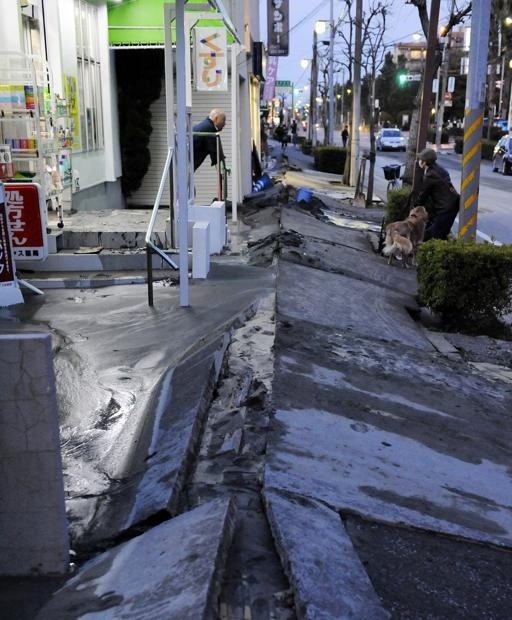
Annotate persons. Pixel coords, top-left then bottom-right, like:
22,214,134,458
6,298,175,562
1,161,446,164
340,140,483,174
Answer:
188,108,226,200
410,148,461,242
340,125,349,148
274,119,298,150
383,120,390,127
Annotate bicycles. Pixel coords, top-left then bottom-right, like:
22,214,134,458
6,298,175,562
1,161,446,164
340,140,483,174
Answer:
381,161,406,202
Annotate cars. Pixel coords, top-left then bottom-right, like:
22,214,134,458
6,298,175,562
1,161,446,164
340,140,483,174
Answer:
376,128,407,152
491,134,512,175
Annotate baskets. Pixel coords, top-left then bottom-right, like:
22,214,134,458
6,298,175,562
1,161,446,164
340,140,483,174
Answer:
383,164,400,180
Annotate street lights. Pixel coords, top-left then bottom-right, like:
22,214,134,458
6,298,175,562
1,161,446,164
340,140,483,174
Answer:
288,15,512,133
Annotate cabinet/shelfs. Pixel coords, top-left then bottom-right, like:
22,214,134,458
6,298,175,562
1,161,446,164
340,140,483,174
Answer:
0,49,66,234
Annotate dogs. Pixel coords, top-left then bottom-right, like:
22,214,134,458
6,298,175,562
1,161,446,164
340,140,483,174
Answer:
381,206,429,269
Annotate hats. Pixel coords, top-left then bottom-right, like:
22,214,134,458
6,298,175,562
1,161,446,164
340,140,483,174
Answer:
416,148,437,162
